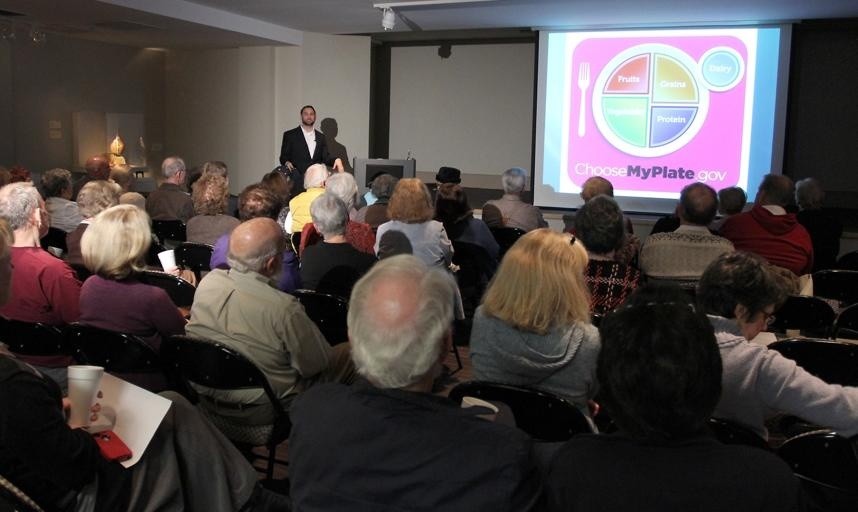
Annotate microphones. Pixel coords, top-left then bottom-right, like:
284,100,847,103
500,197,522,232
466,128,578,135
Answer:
407,147,411,160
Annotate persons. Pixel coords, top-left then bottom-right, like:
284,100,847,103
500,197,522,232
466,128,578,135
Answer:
0,152,858,310
288,253,541,510
184,216,356,409
548,287,807,512
0,218,291,511
693,251,857,441
76,202,188,338
279,106,346,177
0,179,82,325
468,228,601,423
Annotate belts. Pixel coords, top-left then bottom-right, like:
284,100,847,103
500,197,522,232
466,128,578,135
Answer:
204,395,258,410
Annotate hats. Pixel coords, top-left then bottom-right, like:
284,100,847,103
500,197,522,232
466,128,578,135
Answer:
436,167,461,183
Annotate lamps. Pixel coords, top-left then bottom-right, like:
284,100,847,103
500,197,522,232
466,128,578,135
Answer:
378,2,398,38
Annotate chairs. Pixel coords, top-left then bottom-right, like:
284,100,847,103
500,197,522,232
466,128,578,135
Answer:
760,334,858,395
811,261,857,306
774,420,858,512
440,373,595,455
824,297,858,342
641,269,711,315
0,185,524,489
756,279,838,341
604,409,764,456
0,463,45,512
835,246,858,273
233,465,301,511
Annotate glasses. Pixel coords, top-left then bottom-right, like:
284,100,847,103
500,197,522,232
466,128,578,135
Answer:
758,308,776,325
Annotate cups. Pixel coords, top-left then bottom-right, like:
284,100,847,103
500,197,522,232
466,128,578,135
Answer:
47,245,63,257
459,395,499,414
157,249,177,274
66,364,106,427
